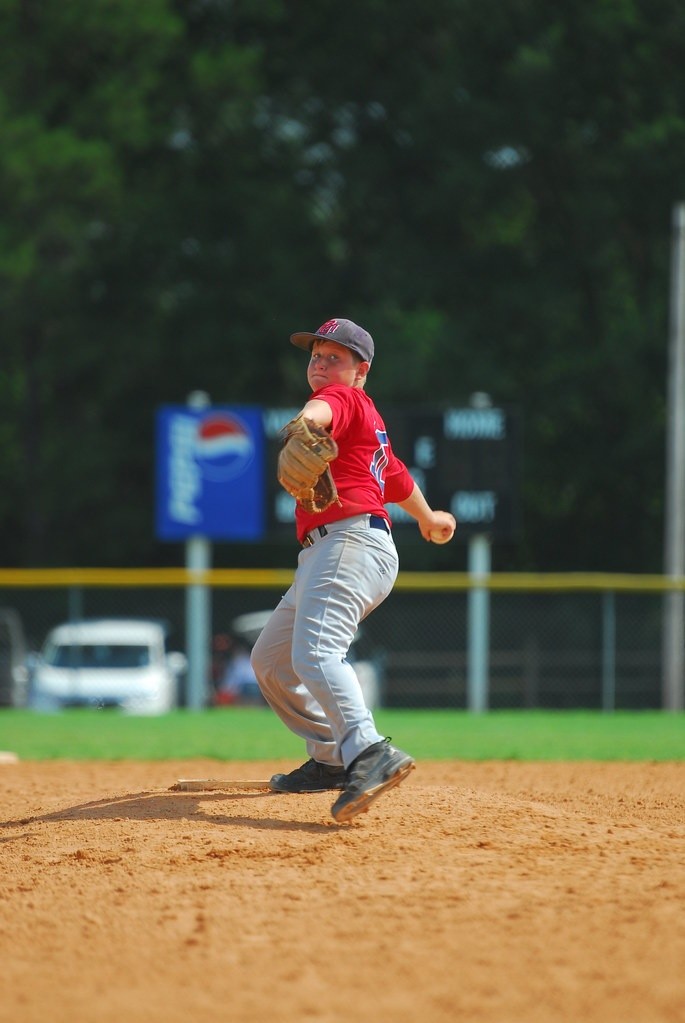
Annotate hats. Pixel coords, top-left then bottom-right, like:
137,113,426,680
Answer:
290,318,375,367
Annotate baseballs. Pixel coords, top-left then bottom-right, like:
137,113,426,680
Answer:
429,529,455,544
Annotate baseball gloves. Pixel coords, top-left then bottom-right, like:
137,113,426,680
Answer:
276,414,343,514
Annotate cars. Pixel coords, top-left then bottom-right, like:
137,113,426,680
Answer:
25,619,189,716
222,610,378,713
0,607,32,708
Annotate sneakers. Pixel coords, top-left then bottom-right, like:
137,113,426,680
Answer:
332,737,415,822
269,758,347,792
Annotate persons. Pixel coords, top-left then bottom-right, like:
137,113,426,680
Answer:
244,317,457,818
